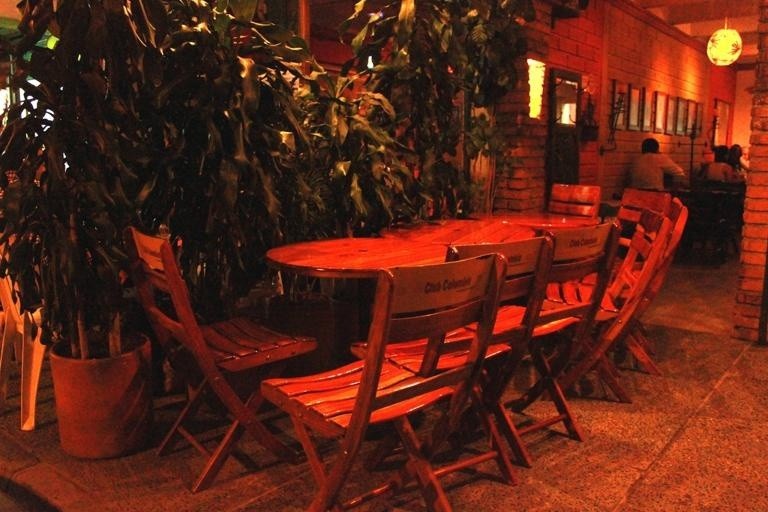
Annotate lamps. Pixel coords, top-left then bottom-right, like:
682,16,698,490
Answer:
707,16,742,66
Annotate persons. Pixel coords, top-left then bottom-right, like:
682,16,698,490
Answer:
703,143,751,184
625,137,686,192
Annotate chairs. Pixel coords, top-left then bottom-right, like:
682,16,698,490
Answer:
351,230,551,487
546,184,601,224
261,253,514,509
566,188,686,400
128,227,314,493
470,219,619,467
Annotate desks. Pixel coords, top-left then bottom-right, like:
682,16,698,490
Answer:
265,210,617,282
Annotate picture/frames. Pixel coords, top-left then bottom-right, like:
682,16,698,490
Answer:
612,79,703,138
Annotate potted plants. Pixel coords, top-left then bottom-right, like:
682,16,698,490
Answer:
1,0,229,458
